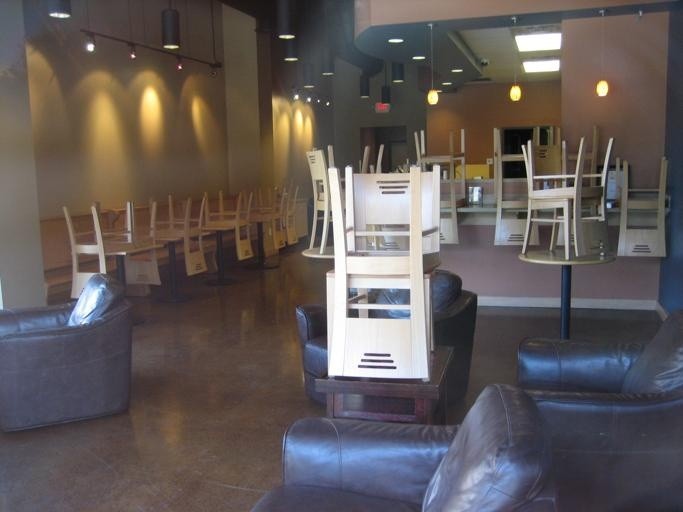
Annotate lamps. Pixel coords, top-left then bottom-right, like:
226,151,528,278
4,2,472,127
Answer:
596,10,609,97
276,0,404,104
509,17,522,103
47,0,72,19
80,0,222,78
427,22,439,106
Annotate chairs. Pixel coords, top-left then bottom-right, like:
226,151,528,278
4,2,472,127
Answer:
1,273,130,434
251,383,557,512
518,311,682,512
295,270,478,414
64,186,300,300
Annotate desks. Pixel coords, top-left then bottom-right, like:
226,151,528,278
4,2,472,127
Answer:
315,347,454,424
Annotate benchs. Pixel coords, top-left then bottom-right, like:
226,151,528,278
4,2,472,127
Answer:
39,196,264,305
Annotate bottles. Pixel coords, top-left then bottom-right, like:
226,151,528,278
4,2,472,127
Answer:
443,169,448,180
